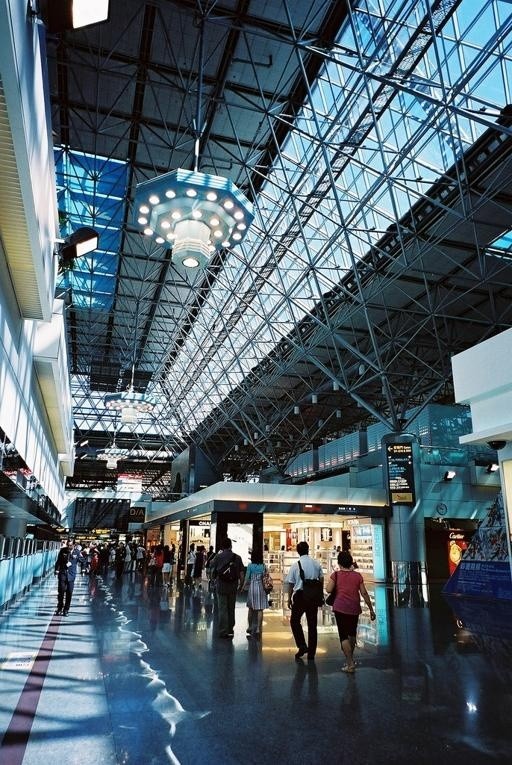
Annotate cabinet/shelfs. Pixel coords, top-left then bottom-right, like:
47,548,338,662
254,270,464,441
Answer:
264,547,374,618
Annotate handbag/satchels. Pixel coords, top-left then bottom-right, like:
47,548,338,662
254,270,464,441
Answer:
218,561,236,581
326,571,338,606
262,565,273,594
303,578,324,607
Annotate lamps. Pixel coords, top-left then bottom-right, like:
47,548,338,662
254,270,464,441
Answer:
265,363,422,450
64,0,111,29
65,225,100,260
132,28,258,273
105,392,125,410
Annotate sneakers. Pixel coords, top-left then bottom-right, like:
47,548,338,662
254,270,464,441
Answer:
218,628,262,639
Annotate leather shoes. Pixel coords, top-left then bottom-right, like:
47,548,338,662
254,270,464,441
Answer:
294,646,356,673
56,609,69,616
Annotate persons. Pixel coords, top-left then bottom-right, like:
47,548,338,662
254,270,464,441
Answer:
339,674,359,722
208,537,243,639
77,575,213,636
74,541,216,596
211,639,235,707
245,641,270,710
327,551,376,673
240,550,273,641
292,657,319,705
284,541,326,661
53,537,85,617
264,539,269,552
